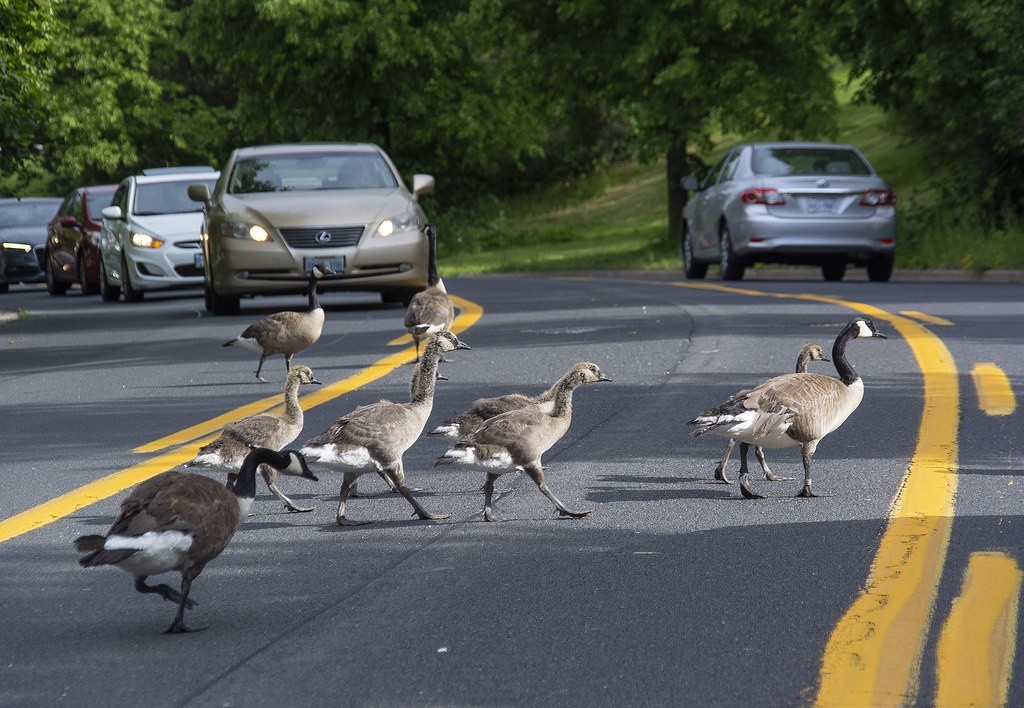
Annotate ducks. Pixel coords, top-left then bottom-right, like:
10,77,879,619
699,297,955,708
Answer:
74,221,892,639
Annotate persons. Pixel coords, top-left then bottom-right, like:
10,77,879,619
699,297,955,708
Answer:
336,159,364,187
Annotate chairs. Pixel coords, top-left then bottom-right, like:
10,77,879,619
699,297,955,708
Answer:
247,168,282,192
825,162,853,175
812,160,829,173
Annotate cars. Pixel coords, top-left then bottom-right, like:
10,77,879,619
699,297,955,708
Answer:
187,142,437,315
678,141,896,282
95,166,240,303
42,184,119,295
0,196,66,294
